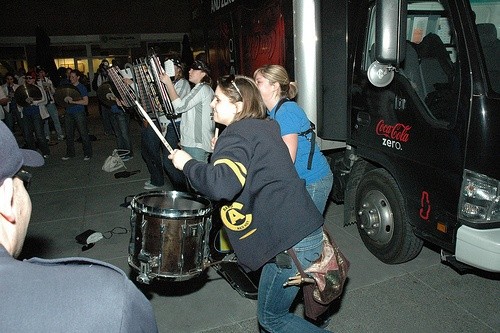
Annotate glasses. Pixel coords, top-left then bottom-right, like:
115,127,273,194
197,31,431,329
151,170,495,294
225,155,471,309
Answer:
226,74,243,98
25,77,33,79
192,60,204,69
14,169,32,190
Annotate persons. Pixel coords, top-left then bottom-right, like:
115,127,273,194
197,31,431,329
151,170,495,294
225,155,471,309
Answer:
0,121,158,333
168,75,334,333
253,65,334,218
0,60,217,201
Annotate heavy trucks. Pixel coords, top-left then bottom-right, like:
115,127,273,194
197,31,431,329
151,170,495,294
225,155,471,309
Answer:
190,0,500,279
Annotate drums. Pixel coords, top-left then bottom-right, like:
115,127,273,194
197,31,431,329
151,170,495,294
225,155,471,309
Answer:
127,189,214,283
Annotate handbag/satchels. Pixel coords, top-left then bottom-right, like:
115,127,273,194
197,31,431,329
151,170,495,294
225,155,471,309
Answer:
301,246,350,320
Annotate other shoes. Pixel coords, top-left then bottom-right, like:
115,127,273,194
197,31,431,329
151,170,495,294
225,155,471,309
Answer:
120,155,129,161
61,155,73,160
46,136,50,143
43,154,49,159
144,179,160,189
58,135,64,140
84,156,91,160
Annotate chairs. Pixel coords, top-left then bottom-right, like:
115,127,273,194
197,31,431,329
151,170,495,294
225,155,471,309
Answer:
478,22,500,92
403,33,453,110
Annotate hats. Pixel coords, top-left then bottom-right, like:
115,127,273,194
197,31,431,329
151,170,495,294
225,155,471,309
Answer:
0,120,44,186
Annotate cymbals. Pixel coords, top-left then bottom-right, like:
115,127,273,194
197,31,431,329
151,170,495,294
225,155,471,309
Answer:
97,82,117,108
53,87,83,109
